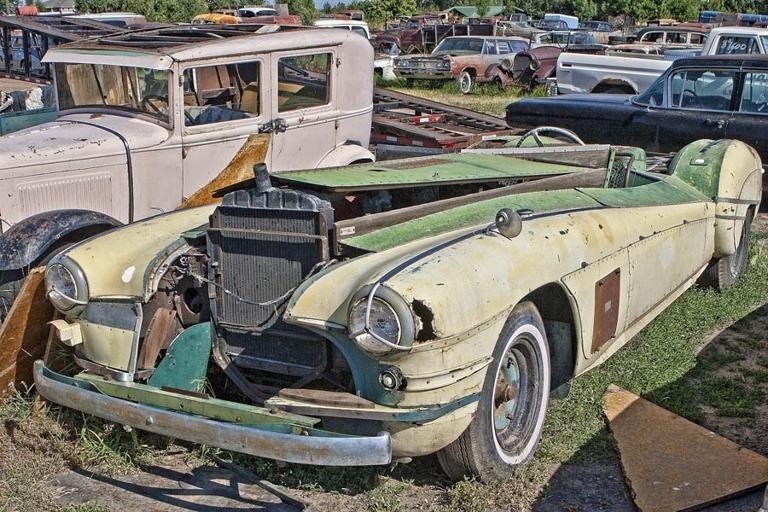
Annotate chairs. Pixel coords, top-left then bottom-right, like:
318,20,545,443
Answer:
196,107,245,124
